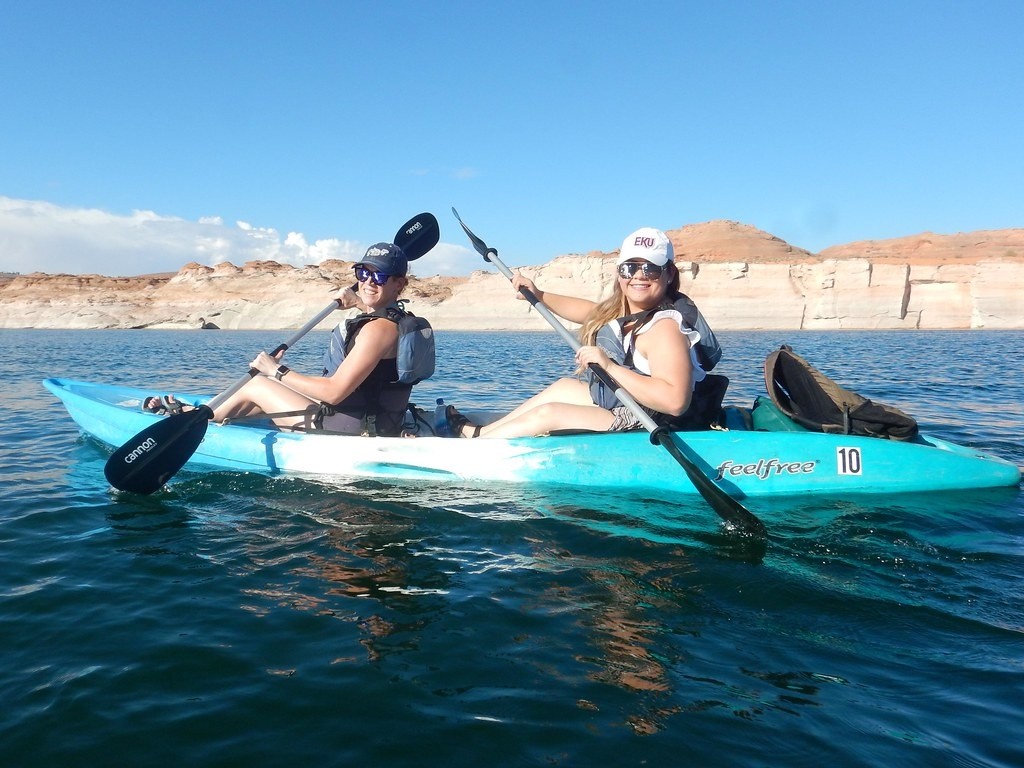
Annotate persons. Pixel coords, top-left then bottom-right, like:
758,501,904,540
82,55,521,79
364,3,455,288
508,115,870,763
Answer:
447,226,705,437
143,242,412,436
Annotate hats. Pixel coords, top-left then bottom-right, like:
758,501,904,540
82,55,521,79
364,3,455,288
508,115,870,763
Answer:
351,242,408,277
617,227,675,266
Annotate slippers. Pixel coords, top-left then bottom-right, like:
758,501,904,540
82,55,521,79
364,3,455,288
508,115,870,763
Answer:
445,404,484,438
162,394,190,416
141,396,171,418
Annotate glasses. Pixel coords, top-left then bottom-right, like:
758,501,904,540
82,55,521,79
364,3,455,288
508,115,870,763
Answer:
617,261,671,281
354,266,391,286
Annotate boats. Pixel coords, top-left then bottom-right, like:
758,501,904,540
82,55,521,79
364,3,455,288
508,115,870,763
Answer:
43,377,1023,501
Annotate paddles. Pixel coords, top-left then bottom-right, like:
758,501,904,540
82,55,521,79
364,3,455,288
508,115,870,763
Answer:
449,204,769,538
103,210,441,496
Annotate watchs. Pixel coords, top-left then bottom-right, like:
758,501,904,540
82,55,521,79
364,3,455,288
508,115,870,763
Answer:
275,365,290,382
